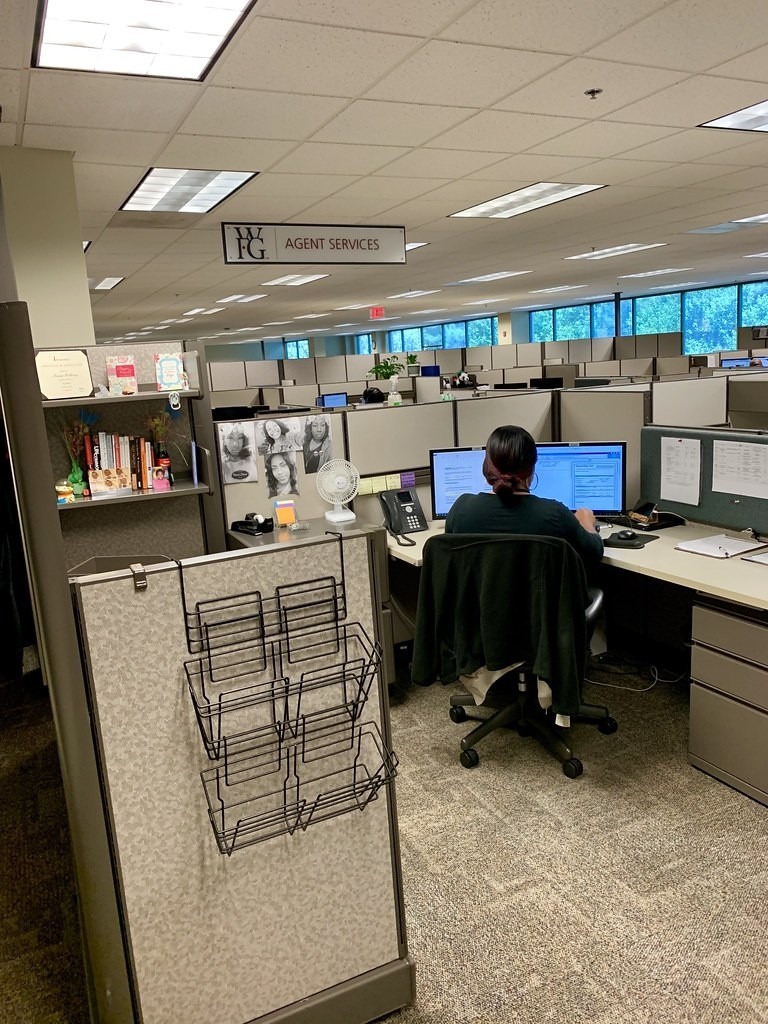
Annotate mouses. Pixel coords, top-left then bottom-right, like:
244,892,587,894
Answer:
618,530,635,541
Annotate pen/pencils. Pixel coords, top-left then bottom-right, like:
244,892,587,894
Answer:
719,546,731,558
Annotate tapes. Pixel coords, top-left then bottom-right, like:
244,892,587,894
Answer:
253,515,265,524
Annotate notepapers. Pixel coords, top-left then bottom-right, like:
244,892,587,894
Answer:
274,500,299,528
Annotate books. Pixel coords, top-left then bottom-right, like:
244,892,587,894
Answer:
84,430,154,491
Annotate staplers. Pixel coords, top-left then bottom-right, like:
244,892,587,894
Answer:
231,520,261,536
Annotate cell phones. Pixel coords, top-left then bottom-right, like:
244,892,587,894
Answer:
633,498,659,517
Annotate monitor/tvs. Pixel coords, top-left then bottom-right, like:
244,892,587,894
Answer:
720,357,752,368
493,376,563,389
429,439,627,521
322,392,348,406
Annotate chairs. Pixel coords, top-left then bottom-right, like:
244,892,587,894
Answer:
409,531,621,775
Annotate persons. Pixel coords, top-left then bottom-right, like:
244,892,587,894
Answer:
360,386,386,404
153,469,171,492
301,414,334,474
219,423,258,483
256,420,303,456
264,452,300,500
749,358,762,369
444,425,604,593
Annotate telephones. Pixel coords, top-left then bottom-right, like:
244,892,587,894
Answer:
378,488,429,534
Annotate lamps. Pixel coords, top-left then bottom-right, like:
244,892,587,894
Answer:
409,306,449,316
562,242,670,262
459,270,535,283
742,251,768,261
95,273,401,344
529,284,588,295
648,281,707,292
117,166,261,216
747,270,768,278
463,297,509,306
28,0,261,84
423,317,451,325
405,241,429,253
696,97,768,135
728,212,768,226
385,289,444,301
618,266,697,280
446,183,609,220
512,303,550,311
464,311,497,319
576,293,616,301
